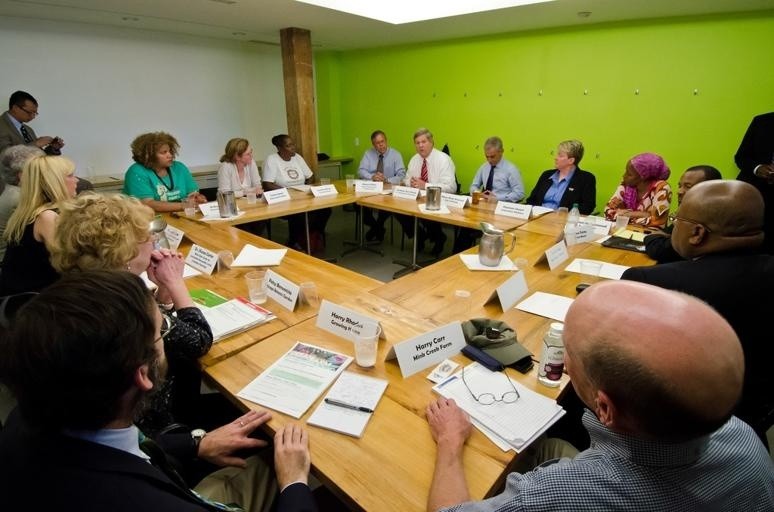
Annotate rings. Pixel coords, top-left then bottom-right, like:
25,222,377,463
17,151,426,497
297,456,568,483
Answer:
239,421,244,426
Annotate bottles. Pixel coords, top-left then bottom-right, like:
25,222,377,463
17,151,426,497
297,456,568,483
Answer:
538,322,565,388
565,203,580,227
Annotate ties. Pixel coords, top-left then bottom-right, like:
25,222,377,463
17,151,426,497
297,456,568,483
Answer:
21,125,32,144
139,429,248,512
377,155,384,174
420,158,428,183
486,164,497,192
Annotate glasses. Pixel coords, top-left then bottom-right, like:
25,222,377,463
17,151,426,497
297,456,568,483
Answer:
668,212,713,234
18,105,38,118
136,230,161,246
461,364,521,405
139,313,178,361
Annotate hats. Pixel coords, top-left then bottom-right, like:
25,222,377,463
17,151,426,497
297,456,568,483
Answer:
461,317,536,367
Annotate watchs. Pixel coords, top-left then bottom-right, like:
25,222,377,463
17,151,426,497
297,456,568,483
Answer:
190,428,206,457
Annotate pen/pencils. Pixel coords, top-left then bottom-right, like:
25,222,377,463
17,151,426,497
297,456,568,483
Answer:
324,397,375,419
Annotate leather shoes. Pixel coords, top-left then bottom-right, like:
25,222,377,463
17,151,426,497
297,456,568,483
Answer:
417,228,428,254
365,225,375,241
430,232,447,258
376,225,385,242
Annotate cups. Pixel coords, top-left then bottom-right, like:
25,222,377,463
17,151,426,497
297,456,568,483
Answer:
352,323,382,367
579,261,600,287
246,188,255,205
426,186,441,210
297,281,319,315
322,177,329,188
215,190,237,218
454,274,470,299
245,271,267,305
615,213,628,230
480,224,516,267
346,175,353,188
182,197,197,216
217,249,235,278
557,206,567,221
472,188,480,206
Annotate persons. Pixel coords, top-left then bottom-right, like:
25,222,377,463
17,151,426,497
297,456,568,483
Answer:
626,180,770,434
1,156,80,315
643,165,722,263
605,153,671,229
49,191,214,428
216,138,263,239
262,134,332,248
0,145,46,262
453,137,524,254
424,279,774,511
0,91,62,161
527,139,596,216
356,131,406,243
734,111,774,229
0,268,319,512
122,131,208,212
395,129,458,255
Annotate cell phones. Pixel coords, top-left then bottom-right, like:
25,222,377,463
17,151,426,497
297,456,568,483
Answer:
52,136,58,144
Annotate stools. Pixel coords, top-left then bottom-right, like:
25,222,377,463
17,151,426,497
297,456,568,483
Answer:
453,224,477,254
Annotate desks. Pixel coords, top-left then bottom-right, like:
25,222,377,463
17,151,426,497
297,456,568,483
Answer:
171,177,403,262
516,209,674,260
203,292,565,510
341,182,556,276
368,228,660,399
46,208,388,374
78,161,344,189
